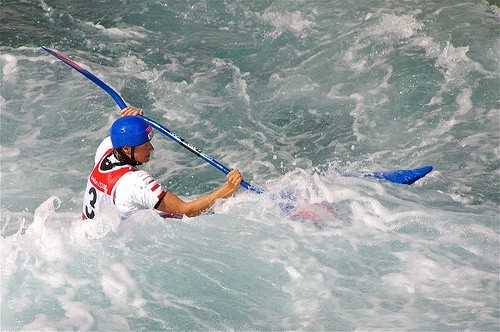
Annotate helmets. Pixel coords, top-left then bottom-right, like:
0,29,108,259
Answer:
109,116,154,149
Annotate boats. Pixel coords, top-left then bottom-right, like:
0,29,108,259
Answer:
186,165,433,225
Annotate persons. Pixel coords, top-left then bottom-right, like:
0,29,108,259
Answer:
82,107,243,220
